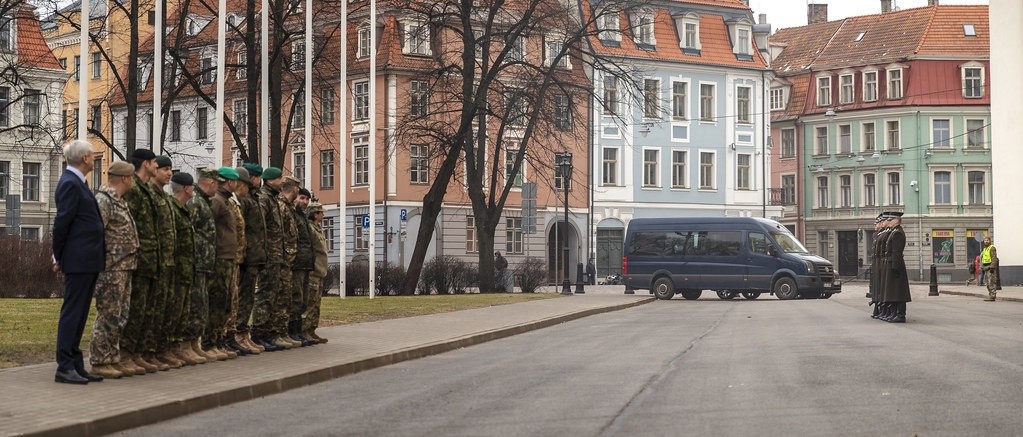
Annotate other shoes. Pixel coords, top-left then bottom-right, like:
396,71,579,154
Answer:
966,280,969,286
983,298,995,301
980,283,986,286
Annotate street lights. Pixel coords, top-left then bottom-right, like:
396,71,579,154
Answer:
559,151,573,295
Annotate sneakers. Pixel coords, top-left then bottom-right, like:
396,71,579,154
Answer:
54,327,327,383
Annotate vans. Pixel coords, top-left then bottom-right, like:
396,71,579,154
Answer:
621,216,842,301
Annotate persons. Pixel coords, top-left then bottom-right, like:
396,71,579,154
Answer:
52,140,106,384
495,251,508,271
981,237,997,301
966,247,987,286
88,149,329,378
868,210,912,322
586,260,596,284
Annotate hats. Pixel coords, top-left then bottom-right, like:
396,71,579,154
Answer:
261,167,281,179
873,212,904,224
242,163,263,177
308,203,327,212
299,188,311,198
107,161,135,176
235,167,253,187
281,176,300,186
217,167,239,180
201,168,225,182
170,172,193,185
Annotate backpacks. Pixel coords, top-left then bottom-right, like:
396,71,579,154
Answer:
969,261,976,274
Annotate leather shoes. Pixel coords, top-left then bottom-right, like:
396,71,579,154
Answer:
870,311,906,322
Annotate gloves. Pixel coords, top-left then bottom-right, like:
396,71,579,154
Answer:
990,269,994,273
